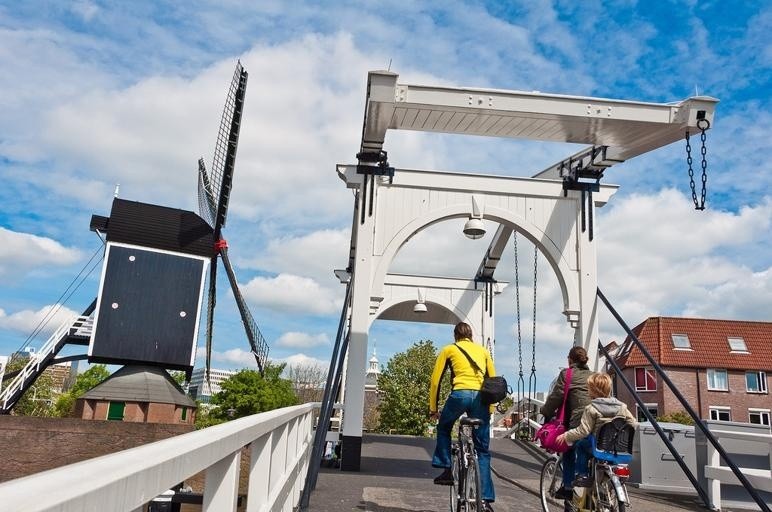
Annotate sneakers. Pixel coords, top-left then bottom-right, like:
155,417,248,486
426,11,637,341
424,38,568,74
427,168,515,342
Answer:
554,487,574,501
481,499,494,512
433,470,453,483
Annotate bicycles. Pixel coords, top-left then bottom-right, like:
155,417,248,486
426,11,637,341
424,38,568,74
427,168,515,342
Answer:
428,412,485,512
536,412,635,512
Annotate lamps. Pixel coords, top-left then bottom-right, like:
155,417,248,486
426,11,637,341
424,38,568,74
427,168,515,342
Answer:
414,303,427,313
463,218,486,240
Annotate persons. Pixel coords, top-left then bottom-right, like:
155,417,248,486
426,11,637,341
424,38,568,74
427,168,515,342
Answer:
552,372,639,488
539,345,602,501
428,321,496,512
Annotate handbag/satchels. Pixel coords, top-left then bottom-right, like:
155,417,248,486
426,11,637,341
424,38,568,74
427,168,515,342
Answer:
534,418,567,451
482,375,507,405
